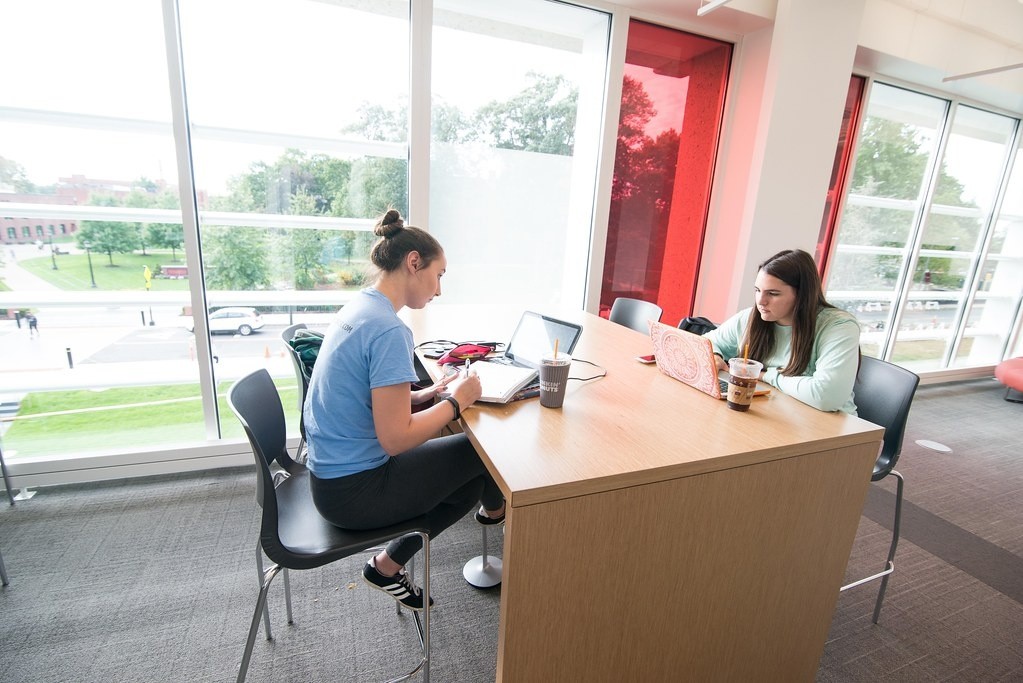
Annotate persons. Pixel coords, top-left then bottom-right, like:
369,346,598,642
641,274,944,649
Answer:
302,208,505,611
26,312,39,334
698,249,863,420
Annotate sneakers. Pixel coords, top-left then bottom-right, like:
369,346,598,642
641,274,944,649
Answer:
474,498,506,527
363,555,434,611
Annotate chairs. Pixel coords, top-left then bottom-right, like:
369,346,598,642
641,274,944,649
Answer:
227,368,431,683
278,322,324,467
838,355,920,624
607,297,664,337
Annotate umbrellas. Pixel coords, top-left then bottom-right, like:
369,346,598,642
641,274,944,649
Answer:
143,266,151,291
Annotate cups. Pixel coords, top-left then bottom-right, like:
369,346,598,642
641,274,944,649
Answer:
539,352,572,408
727,358,763,412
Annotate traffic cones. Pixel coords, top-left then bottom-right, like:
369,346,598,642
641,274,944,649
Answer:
263,346,270,359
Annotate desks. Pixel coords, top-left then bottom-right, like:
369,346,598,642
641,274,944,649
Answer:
396,300,886,683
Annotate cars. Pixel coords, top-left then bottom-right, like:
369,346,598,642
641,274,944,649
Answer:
189,306,266,336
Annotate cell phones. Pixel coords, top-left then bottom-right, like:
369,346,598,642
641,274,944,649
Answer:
635,354,656,364
424,350,445,359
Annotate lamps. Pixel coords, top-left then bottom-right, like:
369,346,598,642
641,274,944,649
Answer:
697,0,733,17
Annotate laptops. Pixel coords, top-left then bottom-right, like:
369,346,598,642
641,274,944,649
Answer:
646,319,771,400
453,310,583,391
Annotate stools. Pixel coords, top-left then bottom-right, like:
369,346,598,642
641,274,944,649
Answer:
995,357,1023,403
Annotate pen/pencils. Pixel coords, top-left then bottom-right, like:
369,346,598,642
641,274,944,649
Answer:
465,357,471,379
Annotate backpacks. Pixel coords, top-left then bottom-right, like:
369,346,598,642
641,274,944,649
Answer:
678,315,717,336
